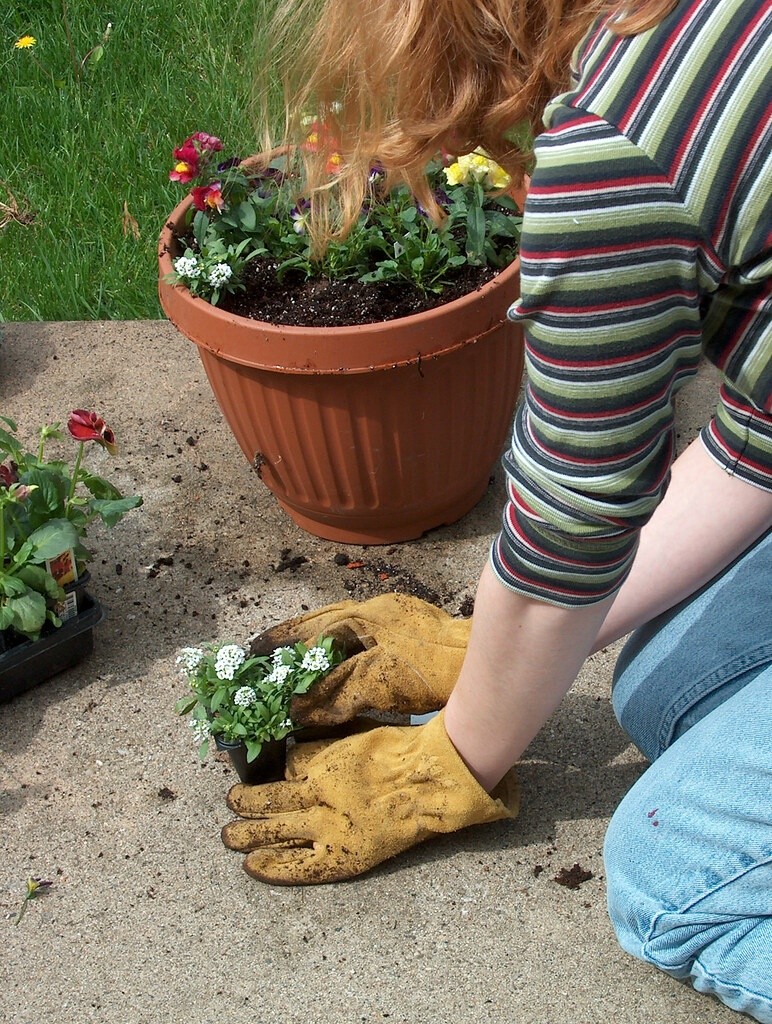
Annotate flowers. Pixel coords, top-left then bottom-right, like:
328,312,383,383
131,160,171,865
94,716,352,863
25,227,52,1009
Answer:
0,408,144,641
160,96,522,307
173,633,345,764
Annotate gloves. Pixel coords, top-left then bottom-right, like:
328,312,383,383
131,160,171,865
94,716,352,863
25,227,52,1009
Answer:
251,592,474,726
220,707,521,886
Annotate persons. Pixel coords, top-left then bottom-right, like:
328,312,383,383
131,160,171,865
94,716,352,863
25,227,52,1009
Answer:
220,0,772,1024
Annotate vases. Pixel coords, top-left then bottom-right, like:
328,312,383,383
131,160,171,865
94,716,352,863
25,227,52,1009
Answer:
205,708,290,786
0,586,103,707
156,145,525,545
